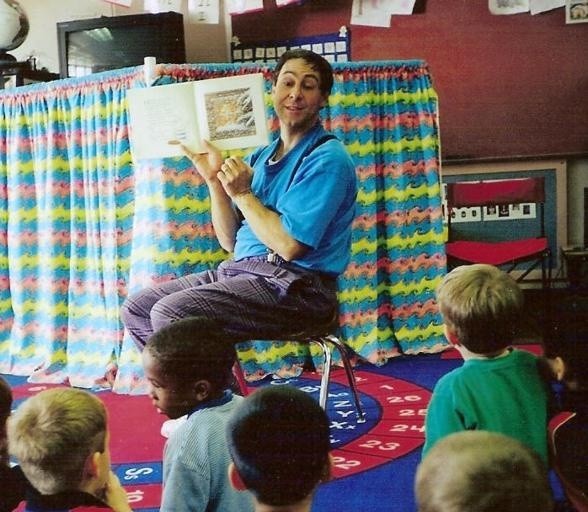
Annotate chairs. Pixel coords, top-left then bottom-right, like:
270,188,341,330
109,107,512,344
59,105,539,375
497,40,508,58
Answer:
443,175,552,335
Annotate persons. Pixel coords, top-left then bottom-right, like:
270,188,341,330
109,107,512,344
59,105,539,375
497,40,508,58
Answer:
5,388,132,512
142,316,259,512
420,264,556,473
414,430,554,511
119,48,359,396
226,386,334,512
1,374,41,512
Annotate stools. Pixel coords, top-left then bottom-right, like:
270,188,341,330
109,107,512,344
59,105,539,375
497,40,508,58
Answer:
231,306,366,425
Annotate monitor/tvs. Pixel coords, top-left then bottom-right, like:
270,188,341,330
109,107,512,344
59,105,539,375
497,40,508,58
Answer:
56,12,186,79
